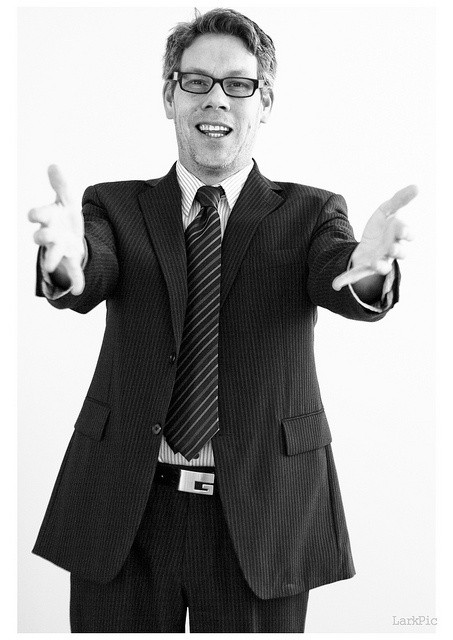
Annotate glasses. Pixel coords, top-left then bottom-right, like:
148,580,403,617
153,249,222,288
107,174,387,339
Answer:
169,71,267,99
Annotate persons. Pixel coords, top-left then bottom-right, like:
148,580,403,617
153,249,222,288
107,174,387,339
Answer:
27,6,421,632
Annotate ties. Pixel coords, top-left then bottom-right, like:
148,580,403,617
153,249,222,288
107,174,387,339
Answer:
165,186,223,460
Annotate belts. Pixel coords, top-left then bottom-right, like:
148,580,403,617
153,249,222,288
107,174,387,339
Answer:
154,463,219,497
